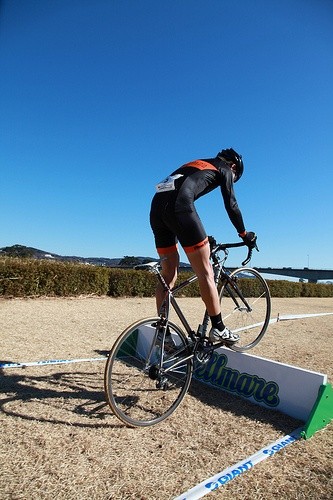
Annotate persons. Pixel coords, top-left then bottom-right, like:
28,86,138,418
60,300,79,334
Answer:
150,149,257,348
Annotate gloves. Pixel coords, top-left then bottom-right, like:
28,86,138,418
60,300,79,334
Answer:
208,235,216,245
238,231,258,248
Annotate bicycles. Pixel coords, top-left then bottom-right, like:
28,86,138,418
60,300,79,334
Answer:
104,242,271,428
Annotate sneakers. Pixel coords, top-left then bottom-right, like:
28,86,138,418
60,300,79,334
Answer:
209,326,241,343
157,333,176,348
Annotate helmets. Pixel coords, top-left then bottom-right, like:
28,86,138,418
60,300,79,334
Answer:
217,148,244,183
